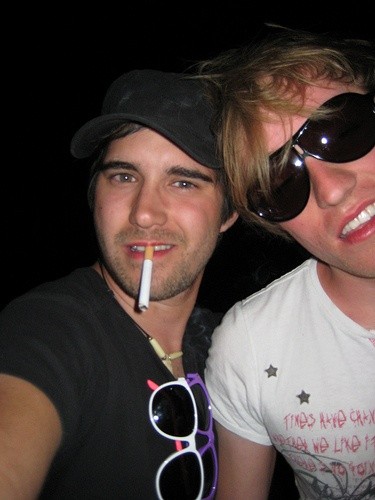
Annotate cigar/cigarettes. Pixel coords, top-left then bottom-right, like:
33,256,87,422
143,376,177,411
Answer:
139,246,154,313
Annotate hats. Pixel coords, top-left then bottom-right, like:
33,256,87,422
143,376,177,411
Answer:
71,70,223,170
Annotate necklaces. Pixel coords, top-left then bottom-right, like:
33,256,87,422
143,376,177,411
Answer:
99,259,184,374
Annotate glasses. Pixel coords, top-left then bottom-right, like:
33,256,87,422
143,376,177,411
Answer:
184,373,218,500
248,93,375,222
147,377,205,500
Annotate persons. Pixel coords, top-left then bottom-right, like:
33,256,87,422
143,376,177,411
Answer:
198,33,375,500
0,68,284,500
